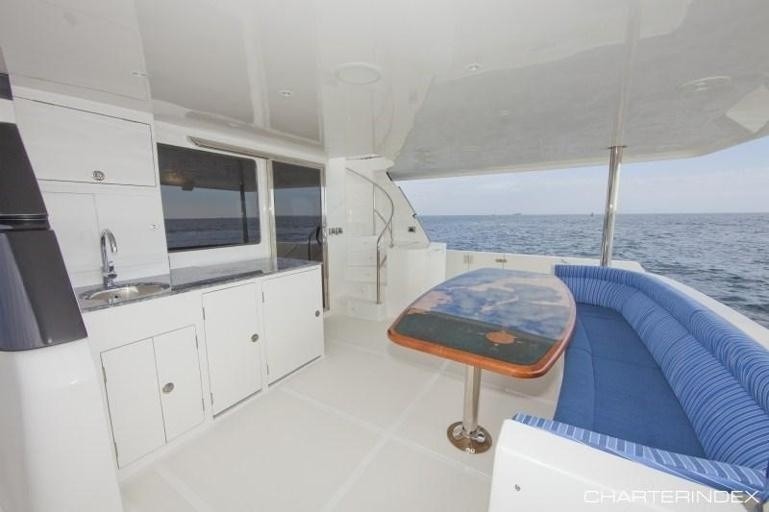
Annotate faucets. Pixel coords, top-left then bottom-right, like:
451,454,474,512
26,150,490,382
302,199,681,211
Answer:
97,228,118,287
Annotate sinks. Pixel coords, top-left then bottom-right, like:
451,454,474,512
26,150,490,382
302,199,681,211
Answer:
77,279,171,309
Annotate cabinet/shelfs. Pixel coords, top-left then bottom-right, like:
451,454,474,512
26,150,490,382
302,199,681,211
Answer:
14,96,169,288
261,266,325,386
199,278,267,421
89,292,208,473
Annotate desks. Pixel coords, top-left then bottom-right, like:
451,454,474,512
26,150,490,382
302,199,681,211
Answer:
386,268,578,454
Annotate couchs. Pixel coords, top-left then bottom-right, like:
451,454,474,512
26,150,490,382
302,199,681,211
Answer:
488,262,769,512
